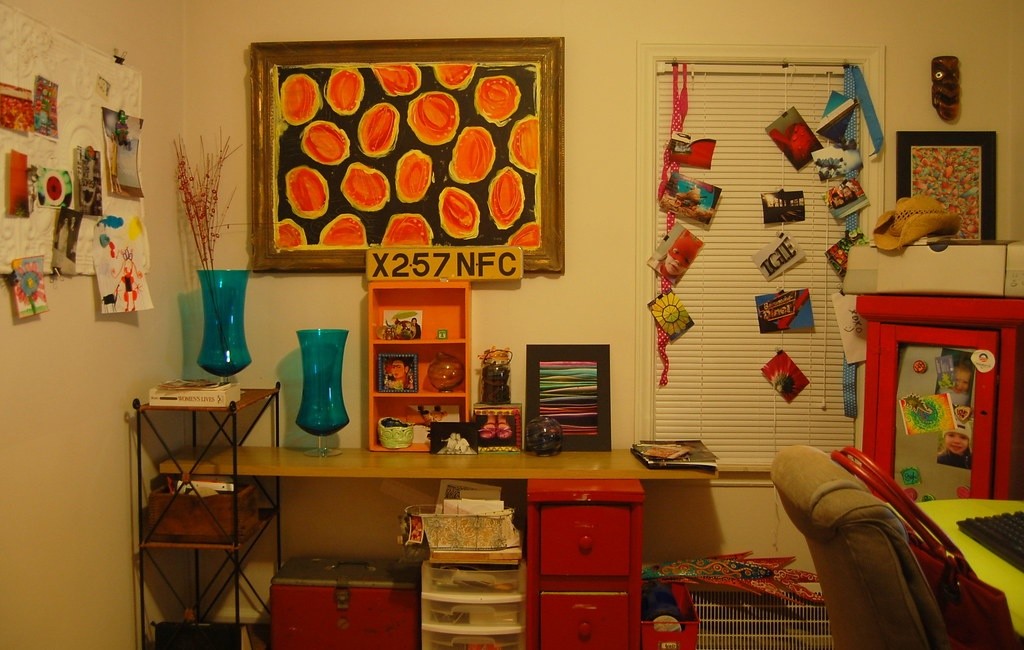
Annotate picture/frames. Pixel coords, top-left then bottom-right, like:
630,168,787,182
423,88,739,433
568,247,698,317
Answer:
524,344,613,451
247,34,567,281
898,129,996,239
377,351,420,392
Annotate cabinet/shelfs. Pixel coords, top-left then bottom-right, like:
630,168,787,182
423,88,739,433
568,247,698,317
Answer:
856,293,1024,502
421,565,527,650
524,481,647,650
366,282,474,454
134,381,284,650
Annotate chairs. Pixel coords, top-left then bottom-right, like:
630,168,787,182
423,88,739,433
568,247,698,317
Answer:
771,445,949,650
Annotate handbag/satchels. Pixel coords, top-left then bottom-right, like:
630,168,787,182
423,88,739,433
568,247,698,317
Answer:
831,446,1018,650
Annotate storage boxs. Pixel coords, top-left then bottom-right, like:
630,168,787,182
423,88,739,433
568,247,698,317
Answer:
271,556,419,650
148,380,241,407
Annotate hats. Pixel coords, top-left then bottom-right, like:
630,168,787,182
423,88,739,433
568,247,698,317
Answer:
873,197,961,250
943,415,972,443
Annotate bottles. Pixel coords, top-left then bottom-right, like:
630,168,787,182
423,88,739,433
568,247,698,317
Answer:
297,329,349,457
526,416,563,456
427,351,464,393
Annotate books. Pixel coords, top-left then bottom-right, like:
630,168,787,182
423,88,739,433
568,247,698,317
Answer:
149,378,241,407
632,440,717,472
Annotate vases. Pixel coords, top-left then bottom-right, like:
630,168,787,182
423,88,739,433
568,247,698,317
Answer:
293,328,351,456
196,268,253,377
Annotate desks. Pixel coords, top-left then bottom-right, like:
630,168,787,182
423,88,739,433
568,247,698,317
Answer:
157,440,719,649
883,498,1024,635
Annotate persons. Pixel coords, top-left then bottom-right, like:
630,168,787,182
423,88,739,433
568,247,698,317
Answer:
938,359,973,407
842,186,858,203
936,416,973,469
831,193,845,209
